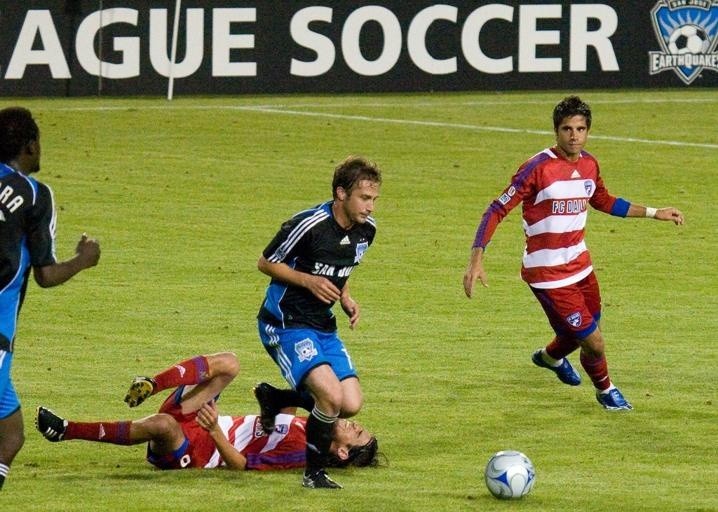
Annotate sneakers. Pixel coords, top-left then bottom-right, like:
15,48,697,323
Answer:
124,377,154,409
302,470,343,490
253,382,276,434
35,406,66,443
532,348,582,385
596,389,632,410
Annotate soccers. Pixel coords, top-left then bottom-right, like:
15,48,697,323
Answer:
485,451,535,500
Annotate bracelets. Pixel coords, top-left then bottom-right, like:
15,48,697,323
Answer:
644,205,658,219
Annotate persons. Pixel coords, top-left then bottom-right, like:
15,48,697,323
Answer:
0,106,99,495
254,156,384,490
464,96,684,413
35,353,389,472
